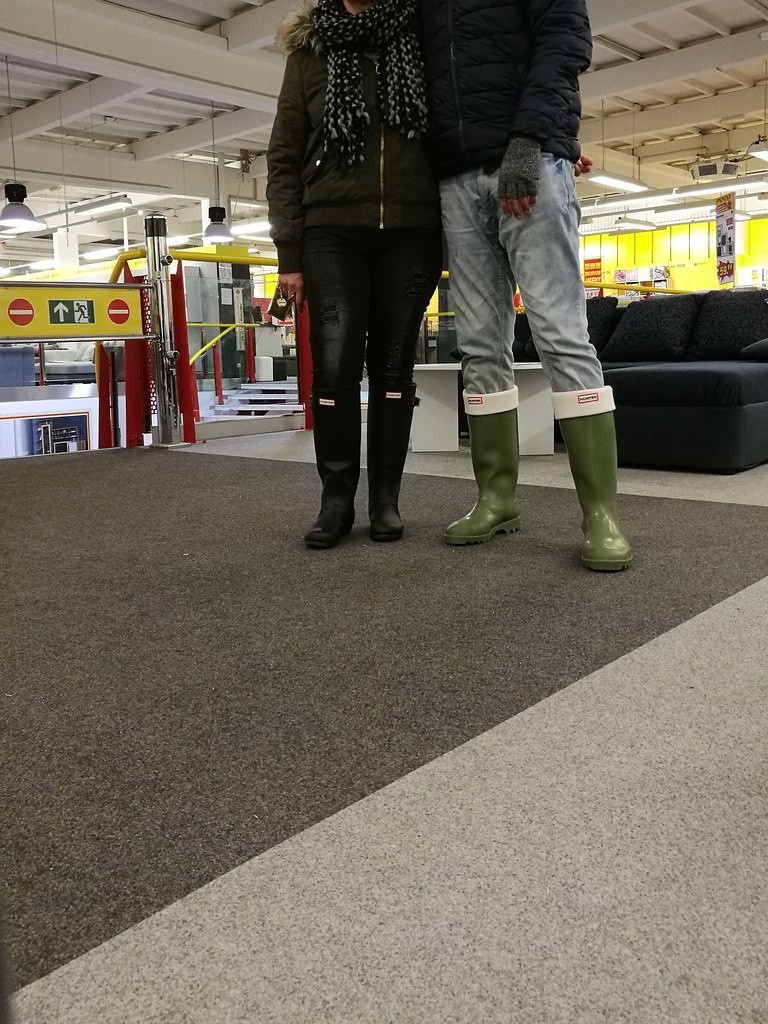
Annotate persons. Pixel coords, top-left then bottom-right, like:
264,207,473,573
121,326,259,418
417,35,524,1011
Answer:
418,0,633,569
267,0,594,547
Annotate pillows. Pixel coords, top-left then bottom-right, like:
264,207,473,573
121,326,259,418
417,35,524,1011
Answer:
525,297,617,358
684,291,768,361
449,340,525,360
604,294,706,361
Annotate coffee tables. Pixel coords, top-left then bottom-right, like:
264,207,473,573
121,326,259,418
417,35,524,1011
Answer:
412,363,554,455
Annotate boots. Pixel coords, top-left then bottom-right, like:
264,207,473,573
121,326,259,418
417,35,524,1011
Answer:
367,382,425,541
303,385,361,549
443,385,521,544
550,385,632,573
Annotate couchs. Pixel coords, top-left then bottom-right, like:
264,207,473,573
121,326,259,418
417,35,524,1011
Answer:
449,288,768,476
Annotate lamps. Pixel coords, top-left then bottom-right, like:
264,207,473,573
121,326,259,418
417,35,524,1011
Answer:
710,153,751,221
202,101,234,242
615,193,656,230
0,197,143,242
748,60,768,161
578,173,768,224
0,55,35,222
587,99,648,191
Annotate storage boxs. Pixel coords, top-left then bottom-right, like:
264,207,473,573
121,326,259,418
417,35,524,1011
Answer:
614,267,672,297
